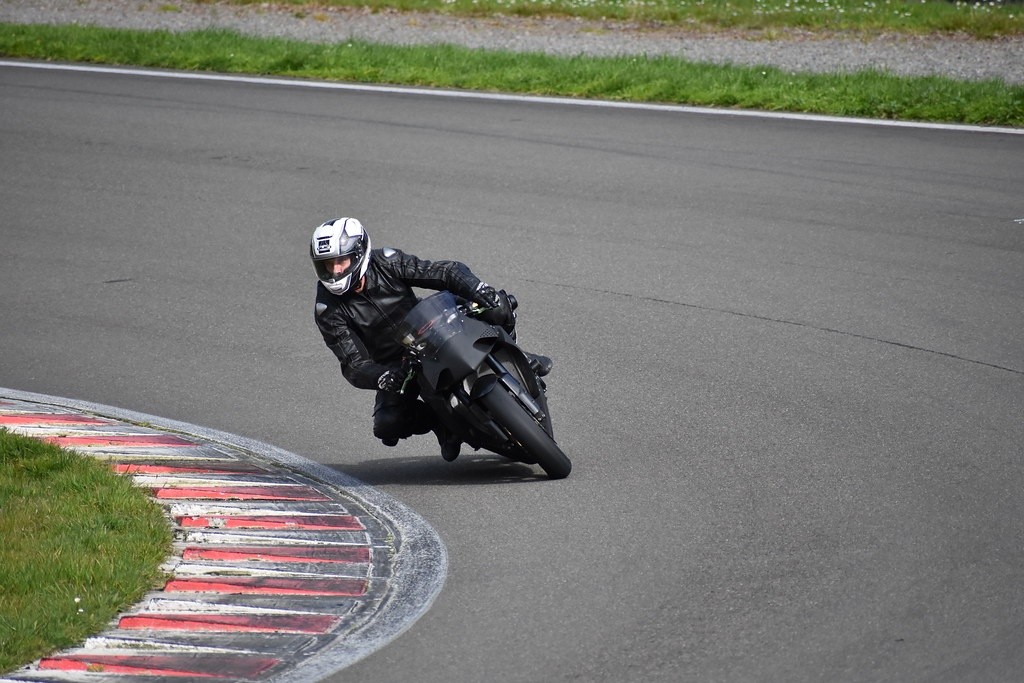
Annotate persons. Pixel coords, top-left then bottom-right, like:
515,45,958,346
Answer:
309,218,553,463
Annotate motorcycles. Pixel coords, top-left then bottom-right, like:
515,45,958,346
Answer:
384,289,572,480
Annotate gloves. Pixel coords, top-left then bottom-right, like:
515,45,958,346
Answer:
376,370,401,394
471,282,499,310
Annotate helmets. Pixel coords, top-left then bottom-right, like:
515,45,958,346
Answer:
309,217,371,296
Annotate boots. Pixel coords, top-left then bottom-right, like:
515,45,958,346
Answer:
507,326,553,377
417,401,461,462
